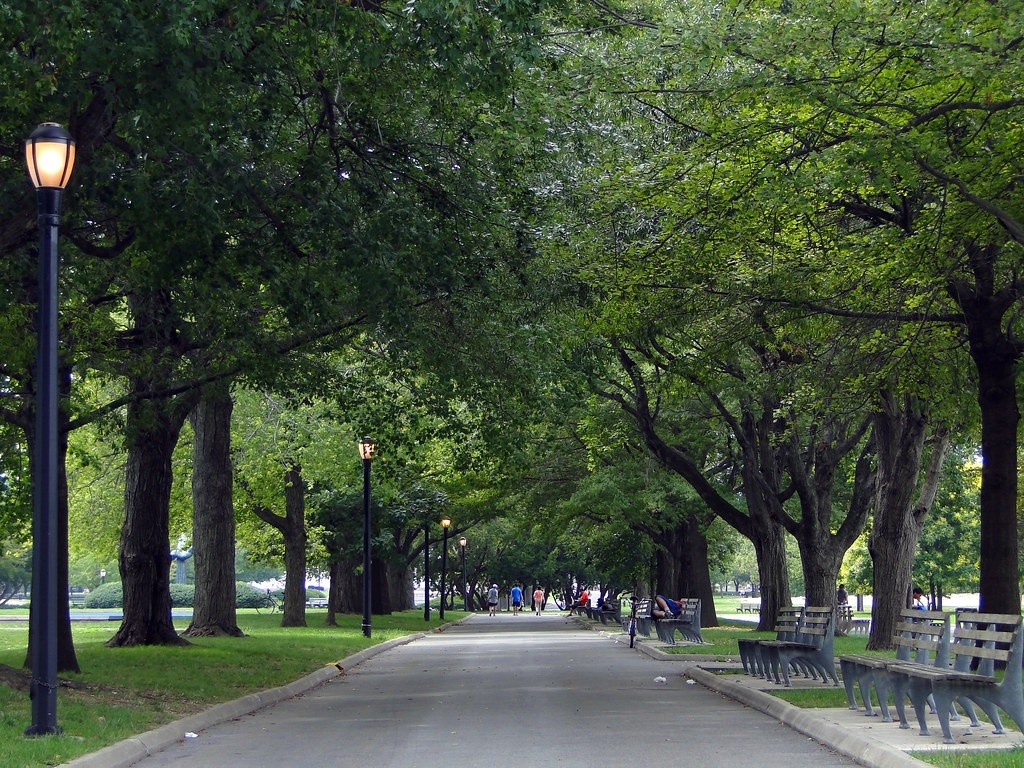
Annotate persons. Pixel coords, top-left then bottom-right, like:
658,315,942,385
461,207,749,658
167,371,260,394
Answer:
511,583,522,616
567,589,588,616
488,584,498,617
599,604,612,611
652,595,683,620
912,587,929,611
533,587,544,616
837,584,848,609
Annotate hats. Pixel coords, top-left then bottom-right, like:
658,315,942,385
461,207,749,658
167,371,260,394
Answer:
493,584,498,588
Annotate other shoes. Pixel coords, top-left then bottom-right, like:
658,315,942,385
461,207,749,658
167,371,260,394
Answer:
494,614,496,617
567,614,572,617
513,613,519,616
489,612,492,617
665,611,675,619
536,612,538,615
539,614,541,616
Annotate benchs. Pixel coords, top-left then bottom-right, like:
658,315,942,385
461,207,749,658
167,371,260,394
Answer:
759,606,842,687
838,604,870,635
592,597,623,625
887,611,1024,744
953,608,978,646
305,597,328,608
912,606,943,641
654,597,704,645
575,599,592,615
737,607,805,679
839,609,962,723
738,603,762,615
620,600,653,636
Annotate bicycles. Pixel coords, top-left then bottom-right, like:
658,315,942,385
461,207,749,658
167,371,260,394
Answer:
255,588,286,616
622,588,639,648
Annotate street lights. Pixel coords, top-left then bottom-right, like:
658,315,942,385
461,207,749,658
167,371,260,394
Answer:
22,121,62,738
459,536,467,612
358,435,375,640
439,516,451,622
